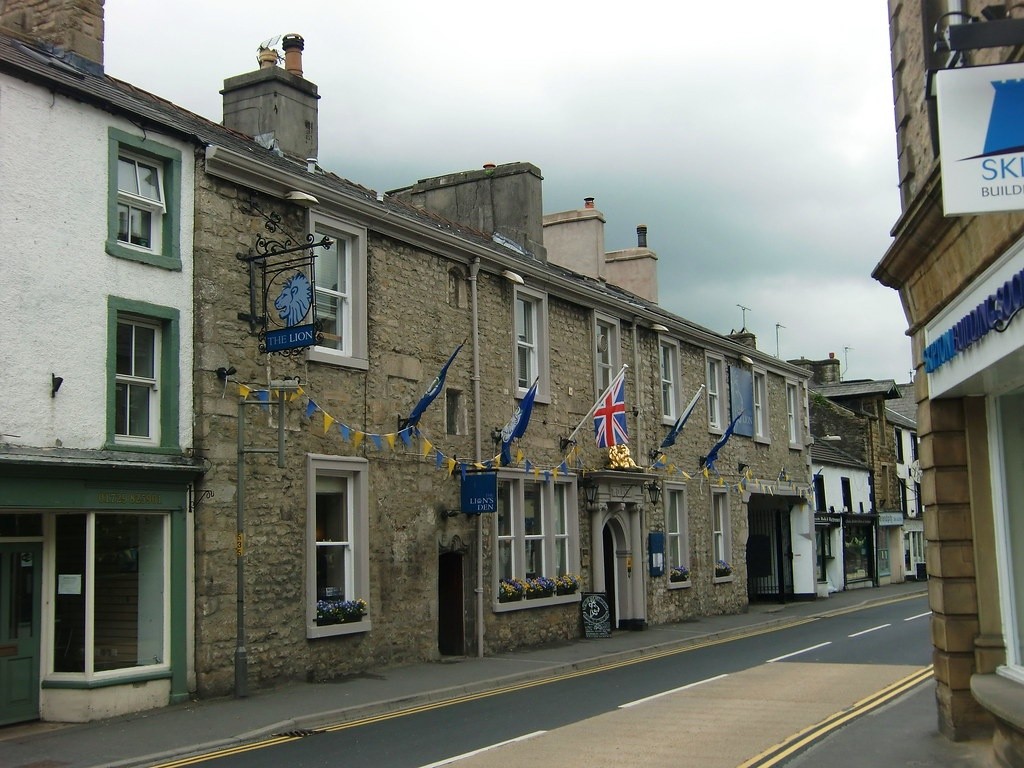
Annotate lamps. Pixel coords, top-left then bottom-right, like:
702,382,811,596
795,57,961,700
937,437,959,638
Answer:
735,354,754,365
283,191,319,208
814,434,841,441
643,479,662,513
577,475,600,508
499,270,525,285
649,324,669,332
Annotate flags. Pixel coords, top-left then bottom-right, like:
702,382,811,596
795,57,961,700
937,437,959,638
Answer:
661,393,702,448
705,414,744,465
408,344,462,424
592,373,629,447
501,381,537,464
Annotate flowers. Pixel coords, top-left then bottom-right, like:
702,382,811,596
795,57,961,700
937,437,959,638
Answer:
715,560,737,572
670,565,689,579
316,599,367,623
499,573,582,598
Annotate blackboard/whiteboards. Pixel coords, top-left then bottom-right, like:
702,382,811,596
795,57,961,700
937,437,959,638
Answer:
915,562,929,581
580,592,612,638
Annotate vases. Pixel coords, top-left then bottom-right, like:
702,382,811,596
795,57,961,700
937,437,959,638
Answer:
554,587,577,596
670,577,687,582
499,593,523,603
716,569,730,577
525,589,553,600
313,613,368,626
